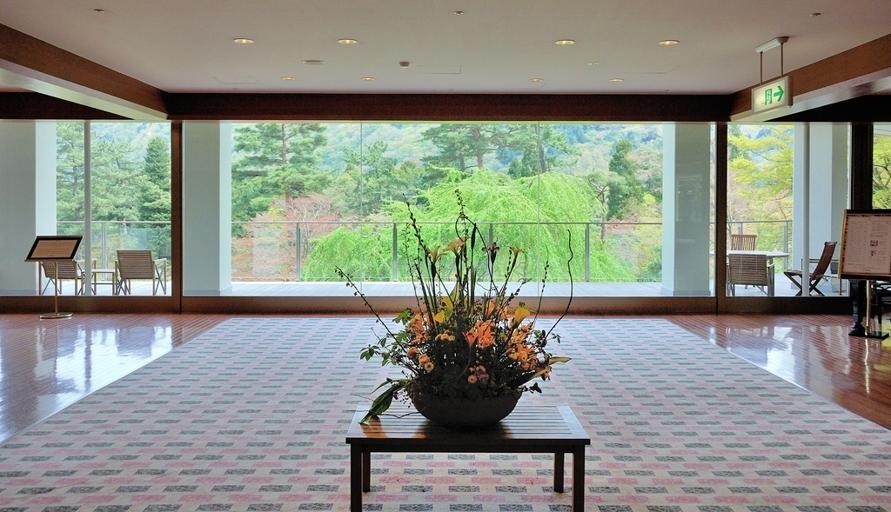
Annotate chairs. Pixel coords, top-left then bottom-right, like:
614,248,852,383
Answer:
783,241,837,297
38,258,96,296
114,250,167,297
726,254,774,297
731,234,757,289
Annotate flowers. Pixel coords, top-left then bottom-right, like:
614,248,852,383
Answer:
332,188,574,425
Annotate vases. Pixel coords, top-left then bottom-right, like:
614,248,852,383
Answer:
405,390,523,427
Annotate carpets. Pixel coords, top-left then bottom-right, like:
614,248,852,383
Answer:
0,317,891,512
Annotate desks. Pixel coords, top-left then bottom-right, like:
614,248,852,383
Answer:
345,403,591,512
80,269,116,297
708,251,789,297
800,258,842,295
